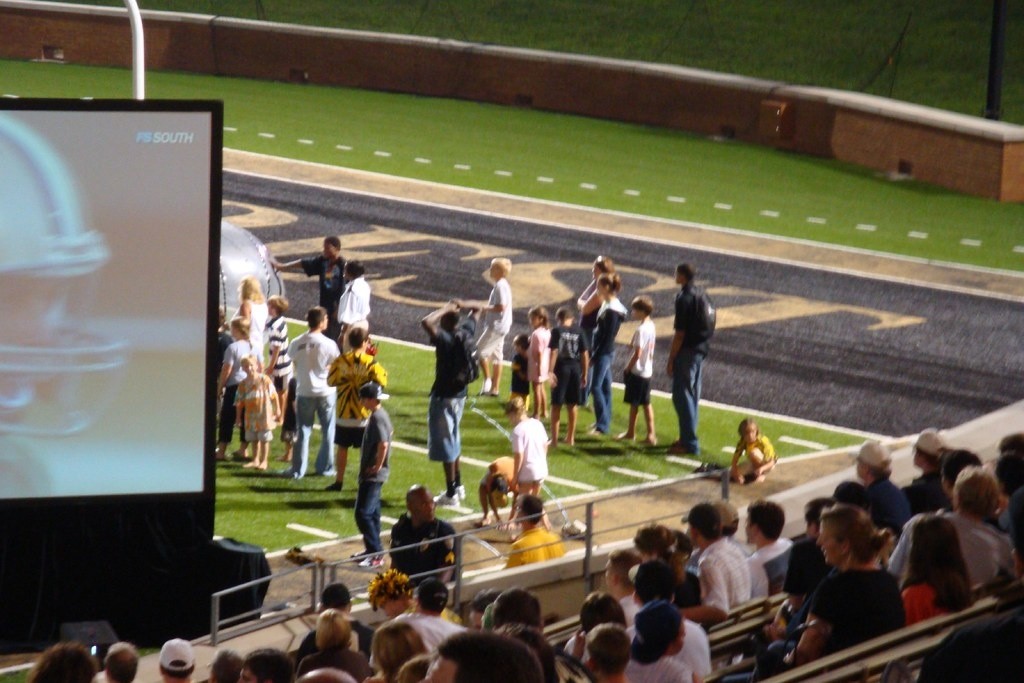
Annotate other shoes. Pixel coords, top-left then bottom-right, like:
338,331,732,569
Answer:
233,453,249,461
611,432,635,441
474,518,491,527
215,451,224,461
640,437,657,446
242,461,261,467
257,461,268,469
432,486,466,505
672,440,681,445
326,483,342,491
667,445,686,453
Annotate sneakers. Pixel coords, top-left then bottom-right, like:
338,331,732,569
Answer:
351,550,369,562
358,555,384,567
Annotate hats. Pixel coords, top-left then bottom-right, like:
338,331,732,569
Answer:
711,500,739,527
627,560,677,596
913,429,947,457
678,503,721,534
359,381,389,400
630,600,683,665
850,439,892,468
414,578,448,613
159,638,194,671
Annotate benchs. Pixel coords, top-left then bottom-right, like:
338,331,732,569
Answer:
539,591,998,683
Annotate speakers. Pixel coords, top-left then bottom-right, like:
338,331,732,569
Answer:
59,621,119,673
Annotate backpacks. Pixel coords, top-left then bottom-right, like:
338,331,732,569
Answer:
680,286,717,342
452,327,479,383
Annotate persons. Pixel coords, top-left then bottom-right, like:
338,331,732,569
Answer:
665,261,716,455
27,235,1024,683
1,113,126,498
